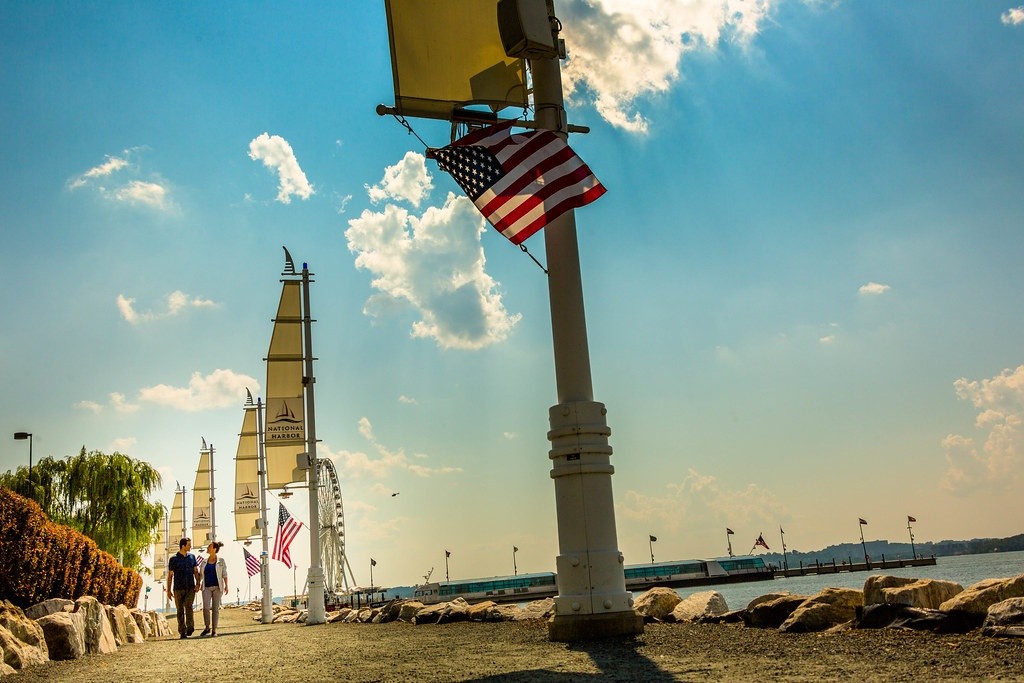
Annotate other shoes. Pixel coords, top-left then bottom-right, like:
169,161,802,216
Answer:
201,628,210,636
212,630,217,636
180,633,187,639
187,625,194,636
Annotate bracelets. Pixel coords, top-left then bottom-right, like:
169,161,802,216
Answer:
196,584,200,585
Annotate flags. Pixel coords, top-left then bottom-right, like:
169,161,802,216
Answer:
650,536,657,541
727,529,734,534
271,503,302,569
197,555,204,567
514,547,518,552
146,586,151,592
908,517,916,523
371,559,376,566
859,518,867,524
145,595,148,599
446,551,450,557
432,114,606,244
756,537,769,549
243,548,261,577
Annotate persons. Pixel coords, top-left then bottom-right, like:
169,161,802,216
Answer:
167,538,200,639
200,542,228,637
768,564,774,571
